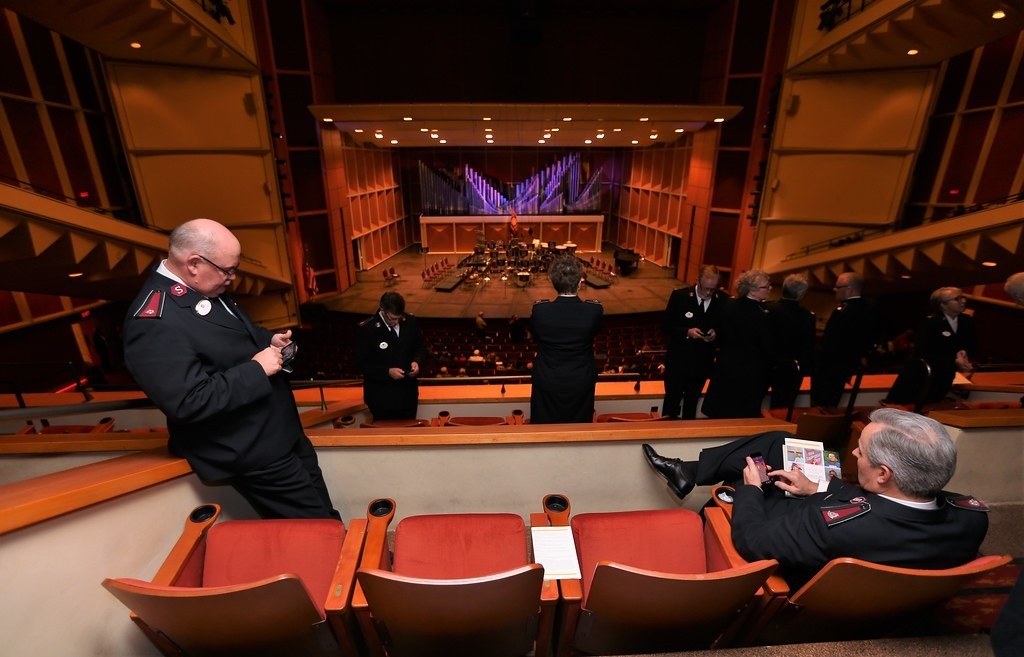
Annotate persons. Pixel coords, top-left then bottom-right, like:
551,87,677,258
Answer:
350,292,432,421
791,450,842,482
661,264,880,418
912,286,982,402
642,408,993,599
123,218,342,520
527,253,604,424
435,311,665,378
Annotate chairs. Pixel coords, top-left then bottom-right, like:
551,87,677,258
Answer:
577,254,619,290
458,265,481,289
710,469,1014,629
350,496,555,657
16,404,661,435
760,400,1024,447
416,325,661,380
421,257,457,290
391,266,401,281
102,503,366,657
541,496,774,656
383,270,396,286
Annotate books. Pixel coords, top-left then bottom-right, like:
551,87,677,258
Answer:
782,444,842,497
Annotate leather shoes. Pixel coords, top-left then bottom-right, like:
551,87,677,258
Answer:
642,443,695,500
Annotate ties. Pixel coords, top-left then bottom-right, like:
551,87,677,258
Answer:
388,326,397,336
208,297,231,313
700,298,705,313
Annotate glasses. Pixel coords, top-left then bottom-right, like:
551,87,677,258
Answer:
699,280,715,292
944,293,964,304
385,312,402,321
199,255,240,276
759,282,771,290
833,285,847,291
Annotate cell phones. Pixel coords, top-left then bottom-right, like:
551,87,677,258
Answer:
401,370,415,375
748,451,774,489
280,340,296,367
